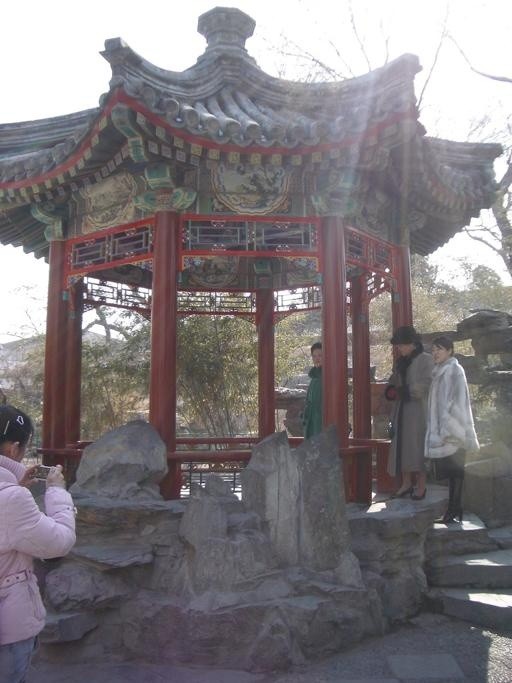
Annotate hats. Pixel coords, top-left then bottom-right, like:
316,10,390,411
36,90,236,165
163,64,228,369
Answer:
391,327,421,344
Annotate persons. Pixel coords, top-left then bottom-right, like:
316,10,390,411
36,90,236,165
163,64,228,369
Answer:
0,404,78,683
299,342,323,441
424,336,481,524
383,324,435,500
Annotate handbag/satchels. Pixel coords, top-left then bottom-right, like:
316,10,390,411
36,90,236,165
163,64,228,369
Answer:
388,421,394,439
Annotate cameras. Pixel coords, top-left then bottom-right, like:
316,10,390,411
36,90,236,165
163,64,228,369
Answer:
33,465,56,483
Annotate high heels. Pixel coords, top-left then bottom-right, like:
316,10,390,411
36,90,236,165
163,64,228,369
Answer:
433,508,462,523
392,486,426,500
